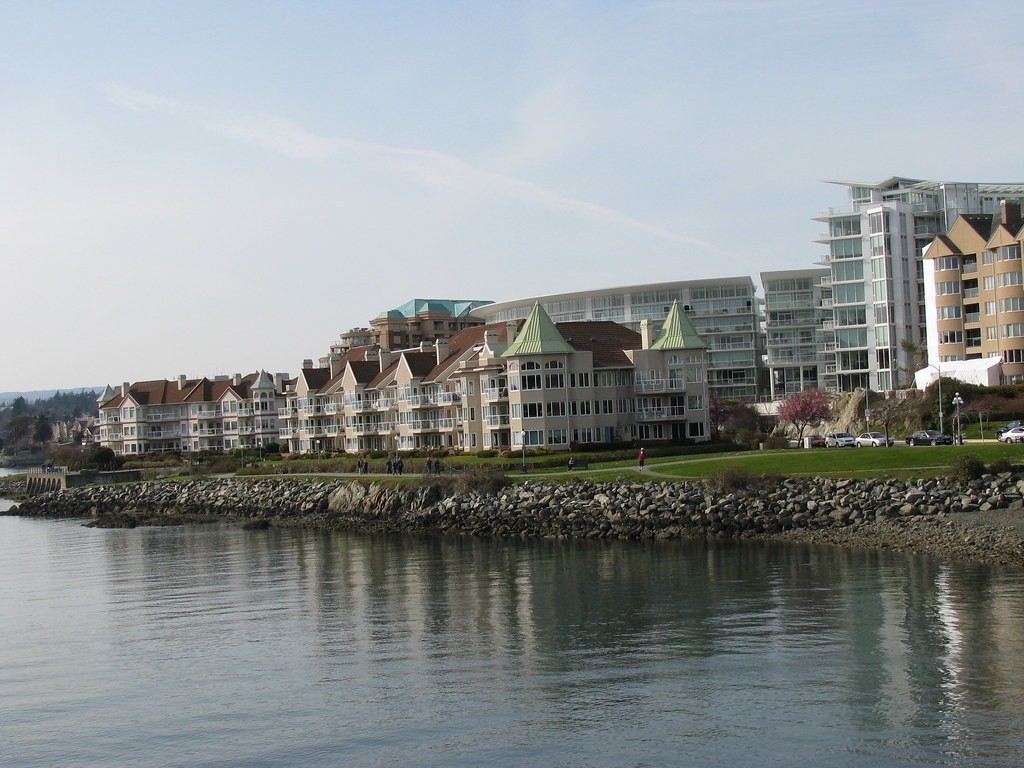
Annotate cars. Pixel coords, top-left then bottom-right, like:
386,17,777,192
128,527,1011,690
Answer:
1000,426,1024,444
801,434,826,448
825,432,856,448
905,429,953,447
854,432,894,447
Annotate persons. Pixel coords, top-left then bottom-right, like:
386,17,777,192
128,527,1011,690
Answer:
434,458,440,475
425,457,433,475
358,458,368,475
569,457,574,464
386,457,404,476
638,448,645,471
42,463,53,472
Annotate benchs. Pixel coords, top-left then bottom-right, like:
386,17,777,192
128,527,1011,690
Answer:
567,459,588,469
42,468,63,474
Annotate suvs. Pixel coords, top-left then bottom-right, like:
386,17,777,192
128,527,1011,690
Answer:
995,420,1024,440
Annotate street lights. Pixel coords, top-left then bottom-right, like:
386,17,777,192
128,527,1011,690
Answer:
394,436,399,461
917,362,943,433
316,440,320,463
188,443,191,453
952,392,965,446
521,430,526,467
259,442,262,459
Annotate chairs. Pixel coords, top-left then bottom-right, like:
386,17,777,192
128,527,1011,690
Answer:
714,328,721,332
735,326,741,330
706,328,713,333
